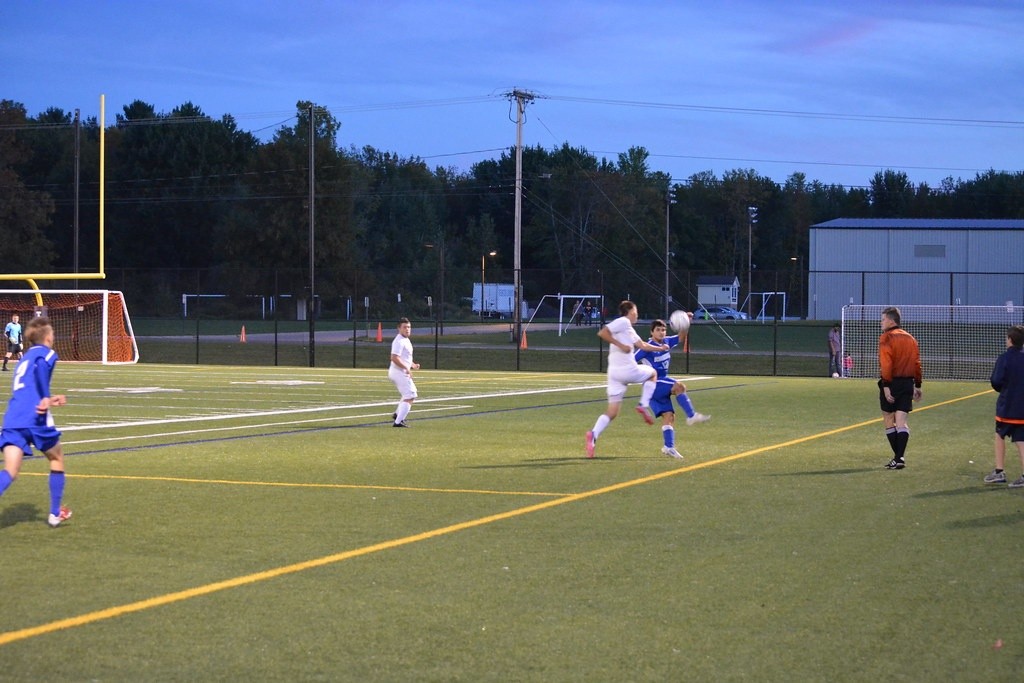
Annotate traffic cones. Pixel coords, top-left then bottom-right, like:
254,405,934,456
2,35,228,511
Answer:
375,323,384,342
520,332,529,350
239,326,248,342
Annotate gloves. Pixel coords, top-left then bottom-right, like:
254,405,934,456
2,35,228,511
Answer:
9,337,17,345
19,343,24,350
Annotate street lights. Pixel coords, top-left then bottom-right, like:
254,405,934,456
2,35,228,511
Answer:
425,242,444,335
748,206,758,320
482,251,498,321
664,184,678,321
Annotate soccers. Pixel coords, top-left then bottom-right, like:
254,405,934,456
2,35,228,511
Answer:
669,310,690,331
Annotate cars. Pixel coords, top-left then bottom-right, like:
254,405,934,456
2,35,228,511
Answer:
692,306,752,320
582,307,601,319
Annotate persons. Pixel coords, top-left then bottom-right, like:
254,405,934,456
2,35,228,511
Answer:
983,325,1024,488
387,318,421,427
584,301,593,326
586,300,669,458
2,314,23,371
0,316,73,527
634,312,711,459
828,322,842,378
877,306,922,470
572,300,584,326
843,351,852,378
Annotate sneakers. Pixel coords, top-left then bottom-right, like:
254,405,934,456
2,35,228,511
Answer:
884,457,906,470
661,445,683,460
1008,474,1024,488
686,412,711,425
984,471,1007,483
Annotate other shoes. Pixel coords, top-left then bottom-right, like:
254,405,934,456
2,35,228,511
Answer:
2,368,10,371
584,431,596,458
392,413,409,422
48,506,73,527
635,403,654,425
392,422,411,427
